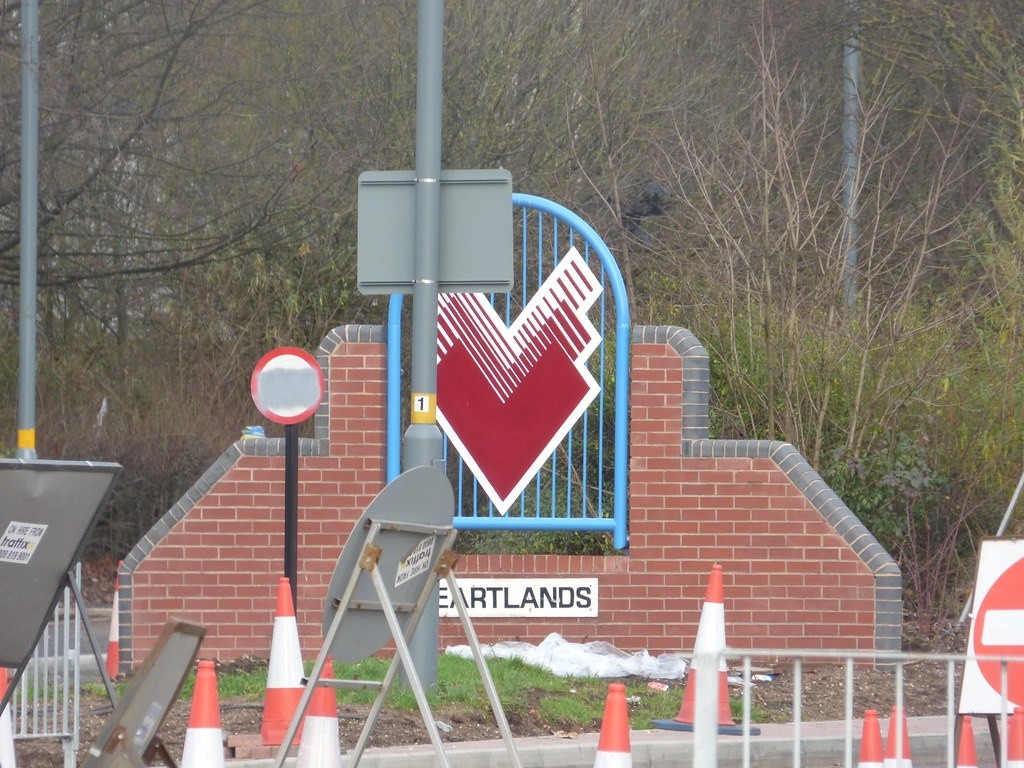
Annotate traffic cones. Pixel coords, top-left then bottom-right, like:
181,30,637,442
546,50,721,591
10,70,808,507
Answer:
258,575,309,746
589,683,635,768
884,705,913,768
952,716,979,768
859,708,889,768
293,654,341,768
178,659,227,768
0,666,17,768
650,564,764,735
103,560,131,678
1005,707,1024,768
